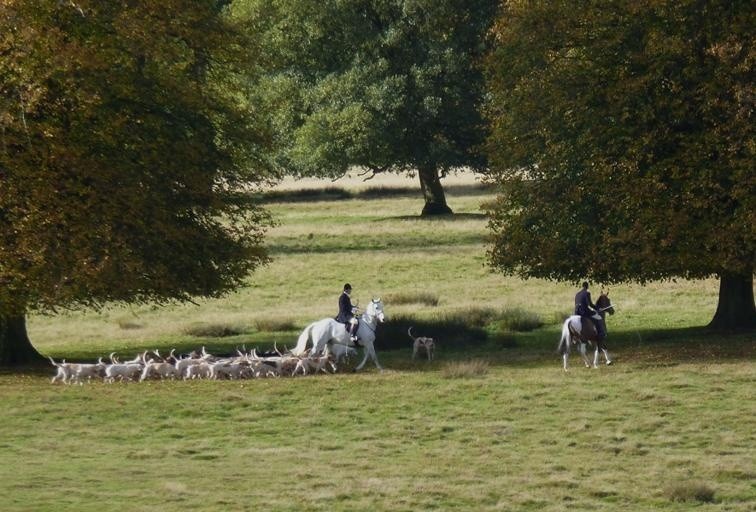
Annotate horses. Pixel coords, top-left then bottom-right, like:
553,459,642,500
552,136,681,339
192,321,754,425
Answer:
290,298,391,370
556,290,618,374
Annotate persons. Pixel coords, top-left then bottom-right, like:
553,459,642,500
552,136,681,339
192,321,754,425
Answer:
574,282,608,338
335,283,361,343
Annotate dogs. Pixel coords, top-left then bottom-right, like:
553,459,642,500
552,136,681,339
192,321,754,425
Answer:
49,341,359,387
405,325,438,365
